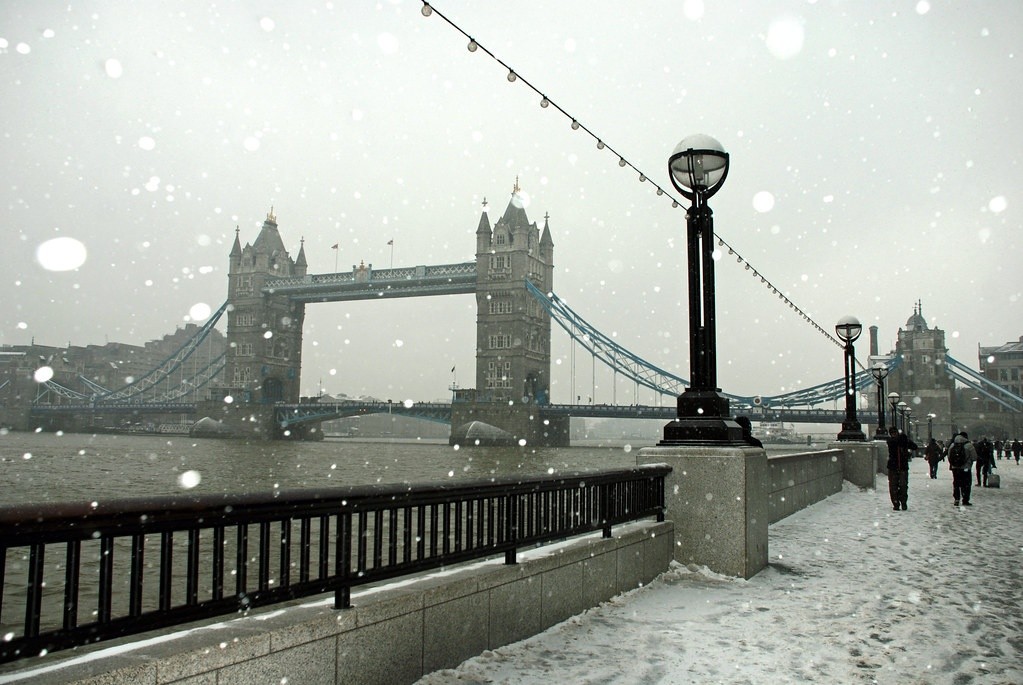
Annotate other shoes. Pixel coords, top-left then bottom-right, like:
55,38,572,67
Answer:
901,498,907,510
954,500,959,506
931,472,937,479
893,501,900,510
983,484,988,487
962,501,972,505
975,484,981,486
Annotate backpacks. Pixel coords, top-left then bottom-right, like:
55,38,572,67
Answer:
949,441,971,467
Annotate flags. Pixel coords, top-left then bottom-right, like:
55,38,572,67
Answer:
387,240,393,245
331,244,338,249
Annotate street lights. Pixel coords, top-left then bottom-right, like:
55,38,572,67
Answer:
655,133,750,448
871,362,912,441
926,415,932,443
835,315,869,443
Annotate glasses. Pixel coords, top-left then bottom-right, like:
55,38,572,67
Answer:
890,430,898,433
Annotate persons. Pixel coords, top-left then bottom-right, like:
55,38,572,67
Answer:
924,431,1023,506
886,427,917,510
